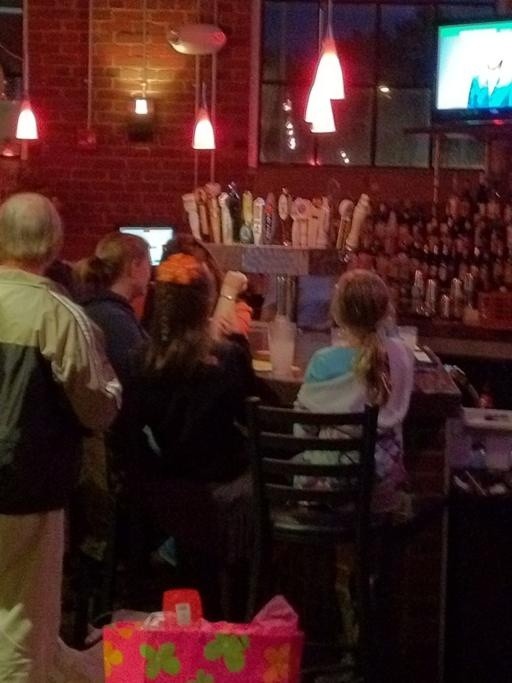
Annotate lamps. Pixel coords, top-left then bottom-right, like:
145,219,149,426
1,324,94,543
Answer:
190,53,218,151
304,0,347,135
14,1,42,141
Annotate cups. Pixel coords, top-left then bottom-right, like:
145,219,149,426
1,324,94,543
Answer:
266,319,297,379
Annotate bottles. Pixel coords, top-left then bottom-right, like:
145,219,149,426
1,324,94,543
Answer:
367,172,512,331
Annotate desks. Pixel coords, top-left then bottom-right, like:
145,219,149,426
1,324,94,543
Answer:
397,315,512,362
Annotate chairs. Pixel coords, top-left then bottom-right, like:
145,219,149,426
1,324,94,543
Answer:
240,399,422,663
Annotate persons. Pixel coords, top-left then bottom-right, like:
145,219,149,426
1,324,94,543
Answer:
0,191,273,683
291,268,418,683
467,53,510,110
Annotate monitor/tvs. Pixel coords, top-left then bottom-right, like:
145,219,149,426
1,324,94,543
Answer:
118,225,175,268
431,18,512,121
